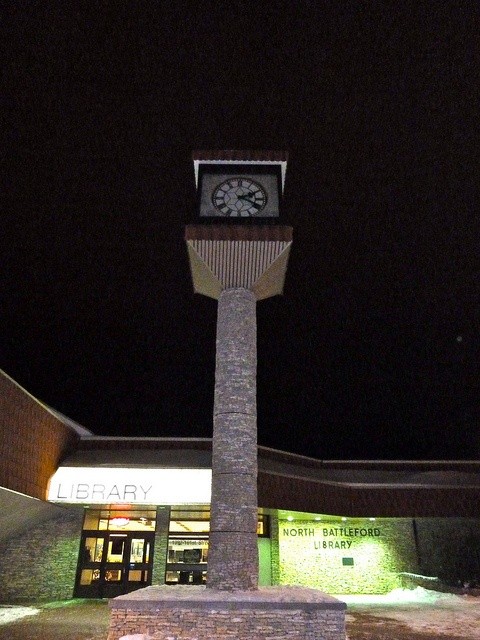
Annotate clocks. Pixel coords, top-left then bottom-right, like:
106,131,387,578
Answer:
211,177,268,217
